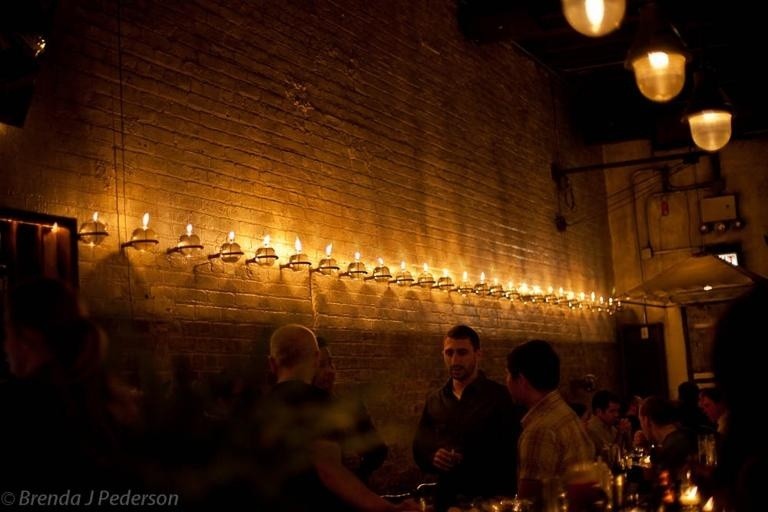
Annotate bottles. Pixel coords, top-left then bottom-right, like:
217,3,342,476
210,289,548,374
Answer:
557,487,570,512
610,446,625,509
588,479,608,512
657,469,678,511
678,469,703,512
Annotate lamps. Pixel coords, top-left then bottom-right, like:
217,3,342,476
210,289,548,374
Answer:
561,0,735,156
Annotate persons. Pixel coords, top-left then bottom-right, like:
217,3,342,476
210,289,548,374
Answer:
504,339,600,509
412,324,523,511
553,379,731,512
702,277,768,511
0,273,106,511
241,322,429,511
308,335,389,511
